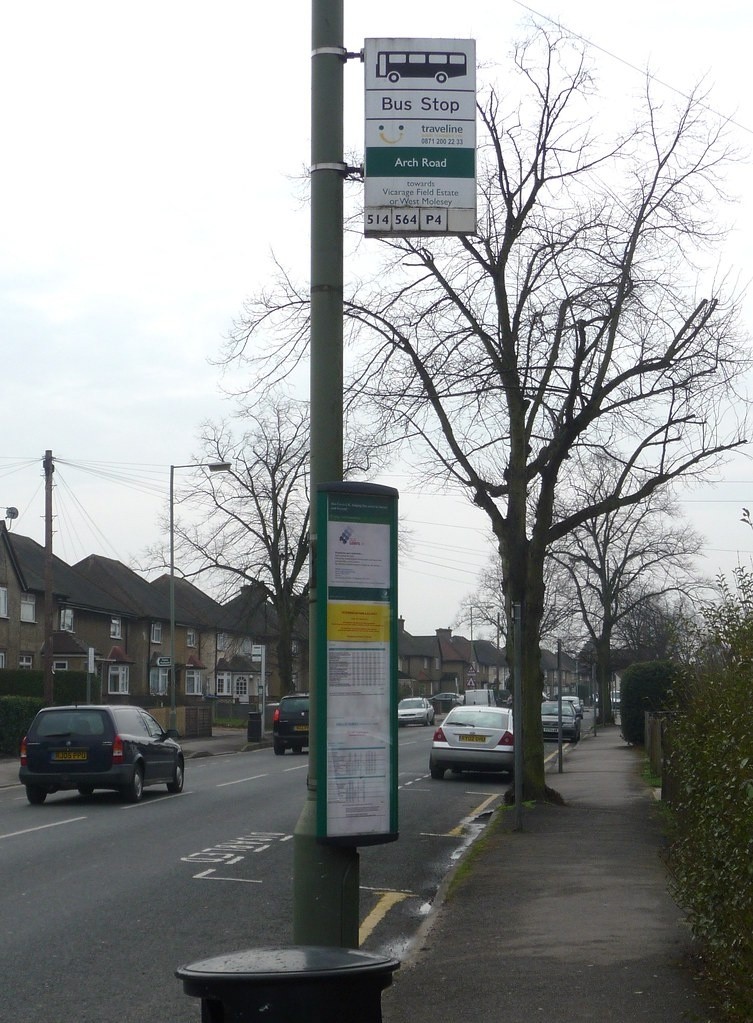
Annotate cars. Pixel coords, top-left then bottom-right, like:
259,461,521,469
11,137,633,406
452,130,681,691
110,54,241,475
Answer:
428,692,465,709
397,696,435,727
495,690,512,706
429,705,514,785
541,701,583,743
561,696,583,720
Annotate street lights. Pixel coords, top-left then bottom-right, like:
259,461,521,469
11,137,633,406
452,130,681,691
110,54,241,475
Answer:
470,605,495,690
166,461,232,732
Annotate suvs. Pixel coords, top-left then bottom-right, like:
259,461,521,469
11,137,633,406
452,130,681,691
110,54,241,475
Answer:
19,704,185,804
272,692,309,756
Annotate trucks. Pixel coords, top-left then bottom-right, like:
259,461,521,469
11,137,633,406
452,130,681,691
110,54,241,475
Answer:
465,689,498,707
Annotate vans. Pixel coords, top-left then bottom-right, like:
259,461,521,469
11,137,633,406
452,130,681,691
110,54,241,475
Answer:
610,691,620,710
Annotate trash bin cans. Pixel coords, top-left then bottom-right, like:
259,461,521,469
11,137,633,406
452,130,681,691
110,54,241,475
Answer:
432,698,453,715
247,711,262,743
174,944,402,1023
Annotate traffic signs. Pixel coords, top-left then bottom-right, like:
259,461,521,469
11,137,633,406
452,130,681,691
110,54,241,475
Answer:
156,657,172,667
